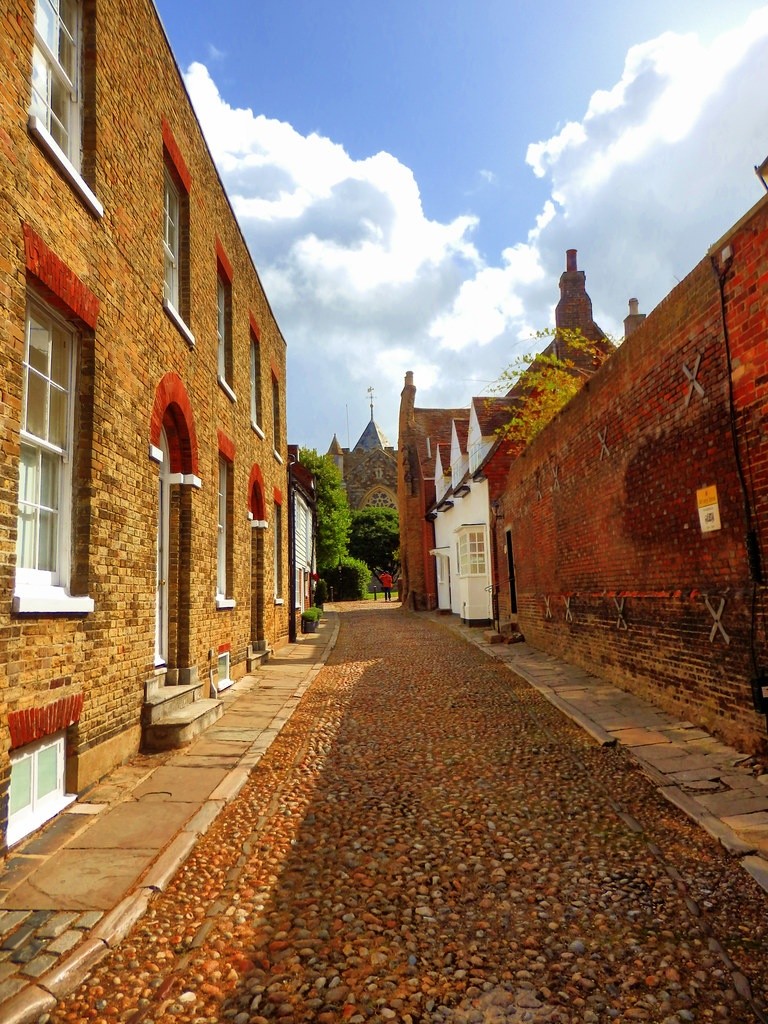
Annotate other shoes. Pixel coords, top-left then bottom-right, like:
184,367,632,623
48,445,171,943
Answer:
385,599,392,602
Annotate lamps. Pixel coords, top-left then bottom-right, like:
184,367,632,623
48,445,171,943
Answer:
424,512,437,522
437,500,454,513
473,474,486,483
453,485,471,498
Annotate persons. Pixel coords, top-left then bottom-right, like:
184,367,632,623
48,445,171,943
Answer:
379,571,393,603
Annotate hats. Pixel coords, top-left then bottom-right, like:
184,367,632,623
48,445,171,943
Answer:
385,571,389,574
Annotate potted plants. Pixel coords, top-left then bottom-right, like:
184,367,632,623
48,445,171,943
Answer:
308,606,324,626
300,611,319,634
